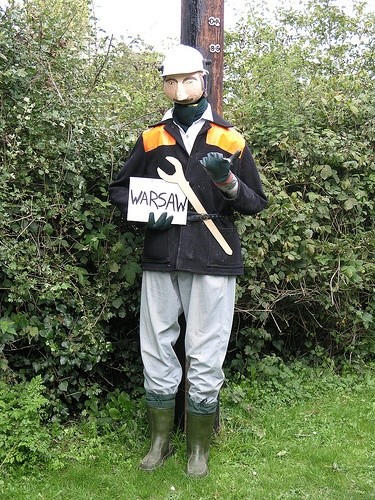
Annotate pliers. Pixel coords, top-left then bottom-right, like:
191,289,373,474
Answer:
157,156,234,256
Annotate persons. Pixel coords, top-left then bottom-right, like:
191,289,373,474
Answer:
107,43,270,477
